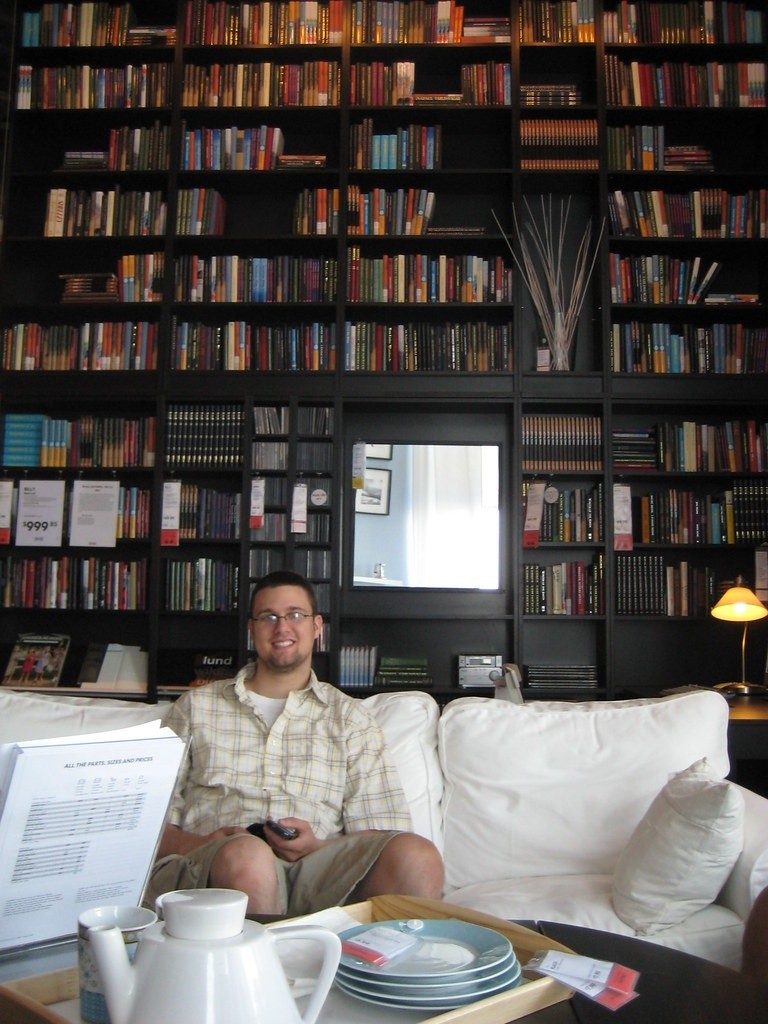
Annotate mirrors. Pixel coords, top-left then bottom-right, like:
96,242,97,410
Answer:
354,441,504,591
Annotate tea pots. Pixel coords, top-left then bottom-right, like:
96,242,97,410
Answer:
87,888,342,1024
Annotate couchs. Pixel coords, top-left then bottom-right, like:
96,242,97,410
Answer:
0,689,767,977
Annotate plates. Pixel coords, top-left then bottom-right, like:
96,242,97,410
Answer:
337,918,522,1011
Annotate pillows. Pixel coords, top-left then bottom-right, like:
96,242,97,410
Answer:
611,756,746,938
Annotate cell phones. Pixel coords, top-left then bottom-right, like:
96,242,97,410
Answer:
267,821,298,841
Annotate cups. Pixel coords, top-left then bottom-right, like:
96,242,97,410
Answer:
78,905,158,1023
155,890,180,920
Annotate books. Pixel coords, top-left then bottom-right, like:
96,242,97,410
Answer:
248,615,332,652
6,3,178,112
527,665,601,692
523,476,768,617
523,411,602,472
351,1,513,107
337,636,435,687
605,119,768,236
518,114,600,173
169,247,514,372
600,0,768,109
517,0,600,106
179,124,340,237
43,120,167,234
0,248,164,471
608,249,768,369
0,476,336,618
166,397,337,470
351,120,489,235
613,414,768,471
180,0,340,107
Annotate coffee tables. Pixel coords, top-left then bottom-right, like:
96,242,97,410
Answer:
0,915,768,1024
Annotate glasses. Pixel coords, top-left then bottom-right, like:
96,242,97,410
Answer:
250,611,312,626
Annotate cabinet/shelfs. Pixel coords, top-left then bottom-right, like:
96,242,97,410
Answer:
1,0,768,695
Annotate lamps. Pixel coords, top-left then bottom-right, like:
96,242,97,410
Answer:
710,574,768,695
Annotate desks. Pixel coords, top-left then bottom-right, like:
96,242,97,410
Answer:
658,689,768,784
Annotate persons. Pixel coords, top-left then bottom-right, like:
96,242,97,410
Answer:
159,575,445,914
4,645,64,685
